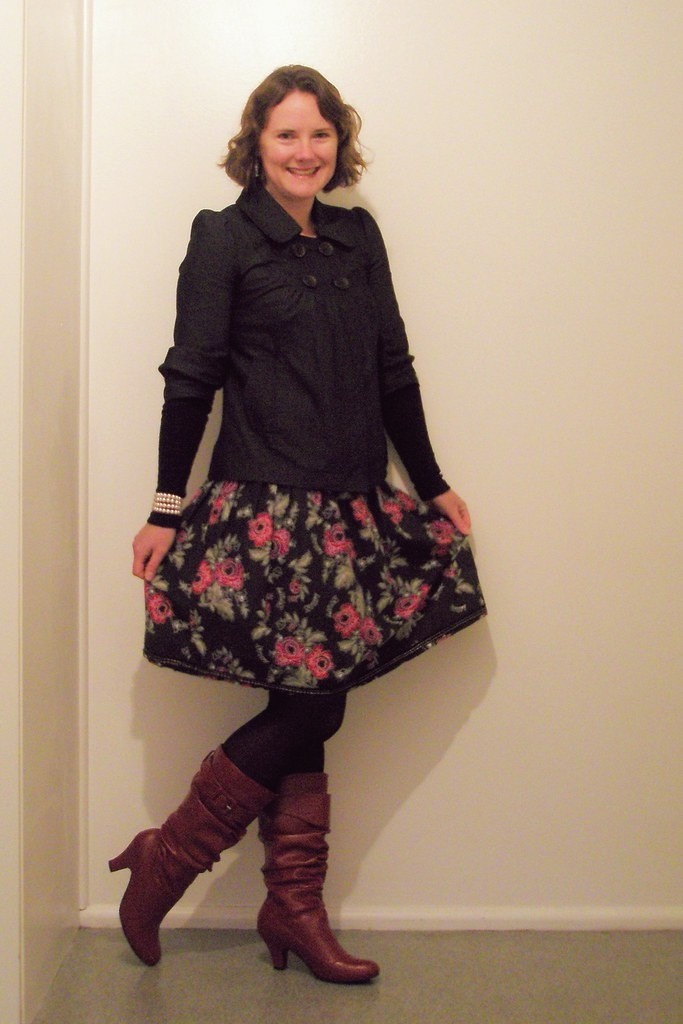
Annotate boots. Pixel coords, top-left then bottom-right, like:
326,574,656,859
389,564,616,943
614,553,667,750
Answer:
110,745,276,966
260,771,380,983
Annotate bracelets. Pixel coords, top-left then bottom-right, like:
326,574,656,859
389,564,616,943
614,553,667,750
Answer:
153,493,182,514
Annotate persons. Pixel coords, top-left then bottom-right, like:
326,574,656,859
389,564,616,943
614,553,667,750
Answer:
109,65,488,982
